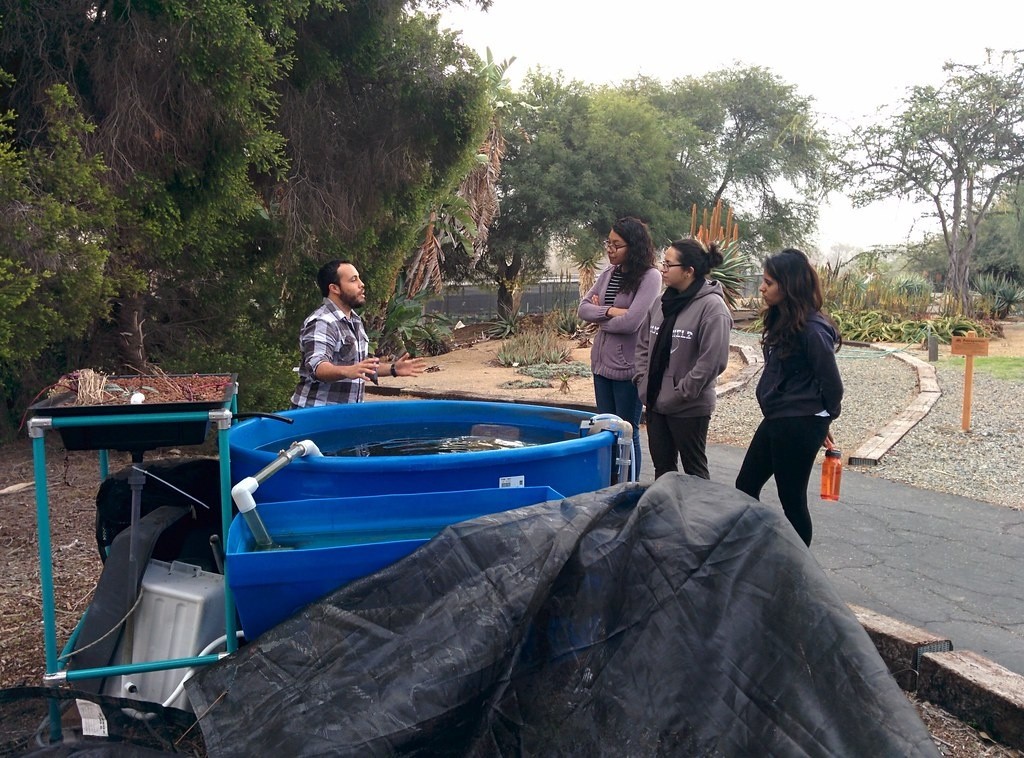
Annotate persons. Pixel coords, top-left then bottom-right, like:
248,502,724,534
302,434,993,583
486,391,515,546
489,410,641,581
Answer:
289,261,427,411
736,249,844,547
577,217,663,483
635,238,734,481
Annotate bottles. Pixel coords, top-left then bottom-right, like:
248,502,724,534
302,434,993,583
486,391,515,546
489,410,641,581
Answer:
821,450,842,501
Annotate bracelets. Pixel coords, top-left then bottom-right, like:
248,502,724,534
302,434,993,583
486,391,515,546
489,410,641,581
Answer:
390,363,395,377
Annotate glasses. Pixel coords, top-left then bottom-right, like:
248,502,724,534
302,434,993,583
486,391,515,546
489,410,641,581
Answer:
604,240,628,252
660,261,684,270
